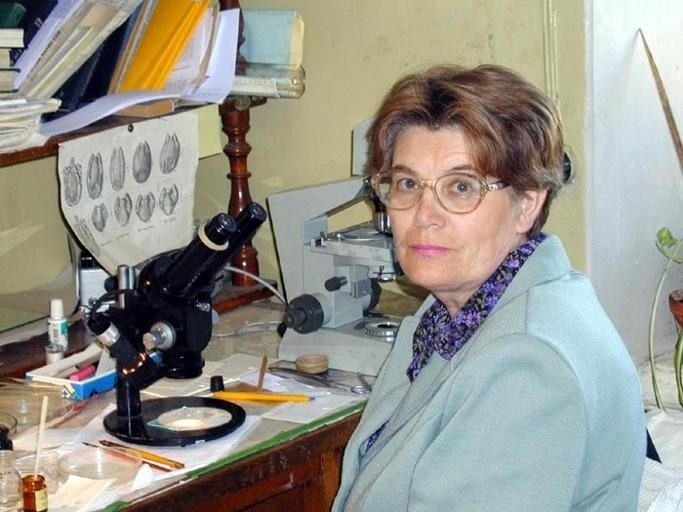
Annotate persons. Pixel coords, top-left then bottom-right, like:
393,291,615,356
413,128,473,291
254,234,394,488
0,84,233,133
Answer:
329,62,647,512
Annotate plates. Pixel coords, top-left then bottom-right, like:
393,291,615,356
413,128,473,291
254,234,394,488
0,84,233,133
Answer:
0,93,277,381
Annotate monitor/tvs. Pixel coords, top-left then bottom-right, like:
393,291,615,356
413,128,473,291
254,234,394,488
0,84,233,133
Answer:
0,0,158,153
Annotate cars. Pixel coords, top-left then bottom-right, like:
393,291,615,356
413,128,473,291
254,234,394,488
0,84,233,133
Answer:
0,411,60,507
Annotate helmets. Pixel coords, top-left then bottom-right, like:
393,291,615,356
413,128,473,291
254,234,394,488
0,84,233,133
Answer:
58,449,143,487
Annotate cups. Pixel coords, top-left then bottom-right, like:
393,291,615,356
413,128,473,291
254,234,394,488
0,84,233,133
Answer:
371,167,512,213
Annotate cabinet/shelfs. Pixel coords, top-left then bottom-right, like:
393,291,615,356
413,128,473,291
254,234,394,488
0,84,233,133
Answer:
22,476,47,512
45,299,69,362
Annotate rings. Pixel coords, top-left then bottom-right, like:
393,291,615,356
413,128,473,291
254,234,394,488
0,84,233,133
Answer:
213,389,315,404
99,440,185,470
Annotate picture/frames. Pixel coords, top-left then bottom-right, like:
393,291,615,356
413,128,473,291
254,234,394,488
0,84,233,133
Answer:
2,275,431,512
